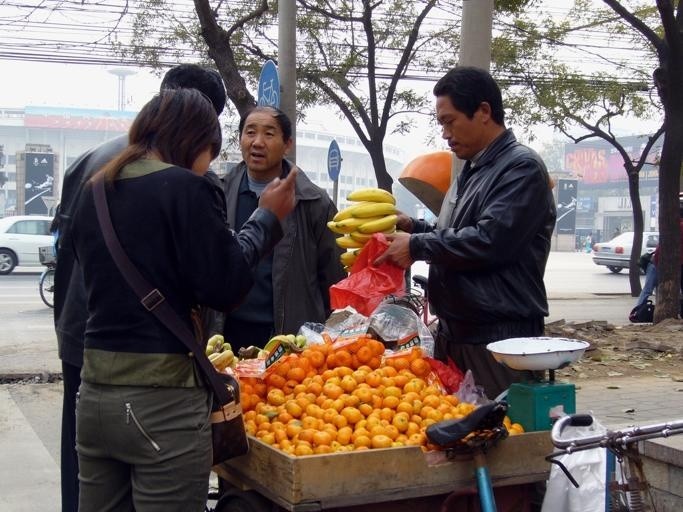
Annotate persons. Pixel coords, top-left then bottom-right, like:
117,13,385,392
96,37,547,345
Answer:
204,106,348,357
638,244,660,306
575,228,621,252
55,63,228,512
68,87,299,512
372,66,555,399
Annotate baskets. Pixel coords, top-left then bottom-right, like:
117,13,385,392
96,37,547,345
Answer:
38,246,57,264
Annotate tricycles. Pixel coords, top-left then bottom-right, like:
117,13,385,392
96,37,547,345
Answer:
212,400,683,511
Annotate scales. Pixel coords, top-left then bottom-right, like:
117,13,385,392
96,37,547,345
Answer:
486,336,590,432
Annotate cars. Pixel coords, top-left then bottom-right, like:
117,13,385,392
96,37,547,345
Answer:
0,214,59,275
593,232,661,274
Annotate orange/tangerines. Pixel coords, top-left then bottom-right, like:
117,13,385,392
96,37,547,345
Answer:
239,338,525,457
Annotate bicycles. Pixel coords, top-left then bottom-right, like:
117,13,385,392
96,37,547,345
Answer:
369,274,440,340
38,247,57,307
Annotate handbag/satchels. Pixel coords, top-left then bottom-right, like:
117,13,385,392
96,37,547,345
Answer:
208,371,250,464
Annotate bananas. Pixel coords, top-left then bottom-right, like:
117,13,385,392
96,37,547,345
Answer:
327,188,399,273
206,334,308,371
